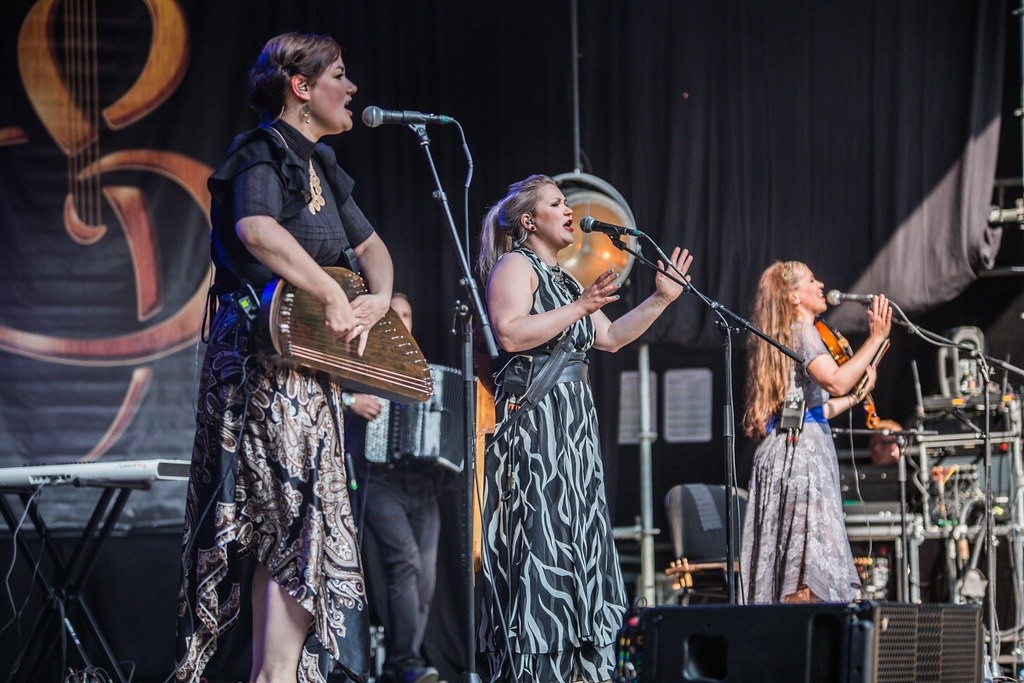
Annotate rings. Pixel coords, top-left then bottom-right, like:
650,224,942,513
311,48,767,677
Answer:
356,325,362,334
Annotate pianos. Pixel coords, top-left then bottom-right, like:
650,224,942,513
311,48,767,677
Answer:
1,457,188,484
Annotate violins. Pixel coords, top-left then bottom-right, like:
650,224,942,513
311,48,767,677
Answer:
814,322,880,430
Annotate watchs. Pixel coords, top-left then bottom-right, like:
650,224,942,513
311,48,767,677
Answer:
344,394,353,409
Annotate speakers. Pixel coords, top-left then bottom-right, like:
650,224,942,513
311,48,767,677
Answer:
641,600,982,683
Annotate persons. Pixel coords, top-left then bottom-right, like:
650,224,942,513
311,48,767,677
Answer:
344,290,442,683
869,420,922,513
478,174,692,682
737,260,892,606
172,34,394,683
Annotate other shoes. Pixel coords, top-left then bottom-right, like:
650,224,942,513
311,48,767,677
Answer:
402,664,439,683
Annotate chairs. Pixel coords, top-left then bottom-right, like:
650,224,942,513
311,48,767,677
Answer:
667,480,747,609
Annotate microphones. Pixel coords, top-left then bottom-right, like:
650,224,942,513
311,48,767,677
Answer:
363,105,454,127
828,289,874,305
580,216,645,238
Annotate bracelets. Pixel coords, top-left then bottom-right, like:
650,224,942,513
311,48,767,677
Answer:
850,393,860,404
